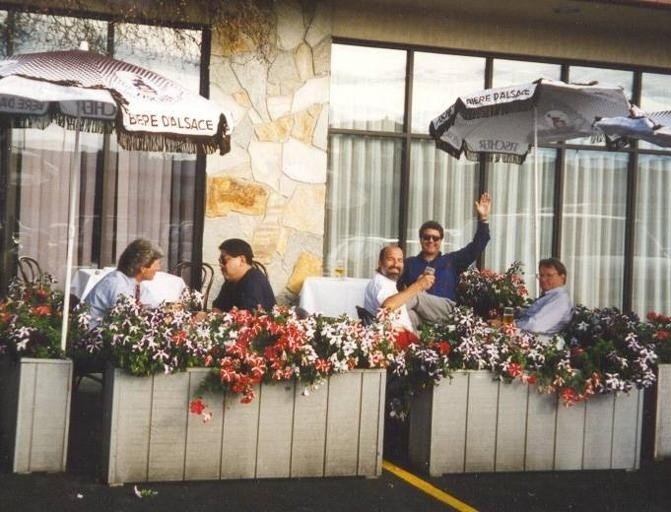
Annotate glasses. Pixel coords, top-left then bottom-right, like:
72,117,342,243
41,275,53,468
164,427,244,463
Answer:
422,234,442,242
218,256,233,265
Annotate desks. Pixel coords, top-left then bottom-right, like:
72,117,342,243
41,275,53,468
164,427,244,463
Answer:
64,269,191,306
293,276,373,324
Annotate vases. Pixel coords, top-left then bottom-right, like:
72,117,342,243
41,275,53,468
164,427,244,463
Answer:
106,364,387,486
643,363,671,461
12,356,72,475
405,350,642,473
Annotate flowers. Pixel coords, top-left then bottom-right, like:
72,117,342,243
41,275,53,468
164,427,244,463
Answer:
456,265,536,319
405,297,671,408
3,265,109,359
92,283,420,424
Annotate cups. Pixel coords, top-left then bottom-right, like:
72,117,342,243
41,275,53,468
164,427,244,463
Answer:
503,313,514,324
423,267,435,276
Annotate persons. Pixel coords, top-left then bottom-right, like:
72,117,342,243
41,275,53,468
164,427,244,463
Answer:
516,258,573,344
79,239,165,326
364,192,491,337
212,239,278,315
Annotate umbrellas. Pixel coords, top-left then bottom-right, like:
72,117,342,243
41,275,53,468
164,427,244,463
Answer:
0,41,235,351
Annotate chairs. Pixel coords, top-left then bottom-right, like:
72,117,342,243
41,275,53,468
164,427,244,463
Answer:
176,260,215,312
17,254,42,285
